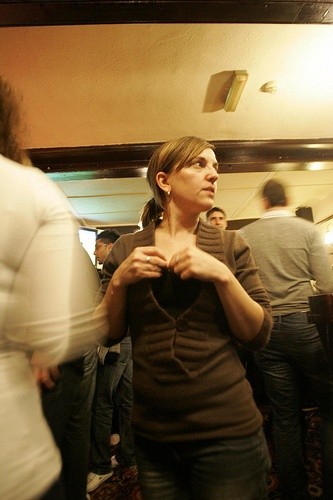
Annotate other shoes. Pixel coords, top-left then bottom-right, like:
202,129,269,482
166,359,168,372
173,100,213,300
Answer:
86,469,113,494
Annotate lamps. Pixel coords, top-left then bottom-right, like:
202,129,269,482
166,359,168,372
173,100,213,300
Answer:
223,69,248,112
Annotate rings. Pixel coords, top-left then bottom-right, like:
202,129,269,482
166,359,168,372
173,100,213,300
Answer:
175,254,179,263
147,255,151,263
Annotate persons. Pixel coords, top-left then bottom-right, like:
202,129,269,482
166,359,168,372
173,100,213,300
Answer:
0,76,97,499
204,205,232,233
88,135,275,500
88,230,134,491
236,180,333,500
